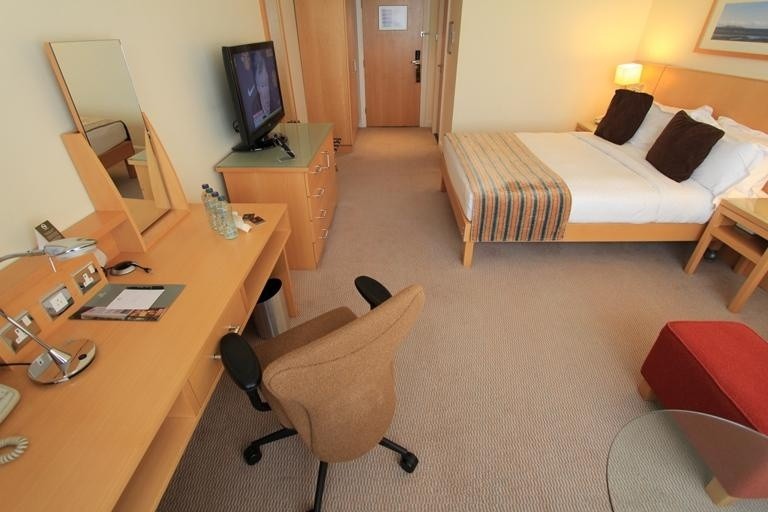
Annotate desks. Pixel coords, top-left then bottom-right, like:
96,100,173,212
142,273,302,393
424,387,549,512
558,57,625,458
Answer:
605,410,768,511
0,204,299,512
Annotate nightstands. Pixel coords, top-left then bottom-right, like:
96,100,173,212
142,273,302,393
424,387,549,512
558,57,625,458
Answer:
576,119,599,132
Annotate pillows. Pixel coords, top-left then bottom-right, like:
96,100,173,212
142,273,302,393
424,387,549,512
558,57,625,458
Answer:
629,104,714,151
594,89,654,145
646,109,725,183
691,139,764,191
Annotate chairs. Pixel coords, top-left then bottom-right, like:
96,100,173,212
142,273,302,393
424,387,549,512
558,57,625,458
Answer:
215,276,426,512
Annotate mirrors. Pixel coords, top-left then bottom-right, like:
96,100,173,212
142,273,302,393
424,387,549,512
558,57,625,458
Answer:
48,40,170,235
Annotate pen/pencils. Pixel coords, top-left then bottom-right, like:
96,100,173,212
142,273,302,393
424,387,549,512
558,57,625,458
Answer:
125,286,165,290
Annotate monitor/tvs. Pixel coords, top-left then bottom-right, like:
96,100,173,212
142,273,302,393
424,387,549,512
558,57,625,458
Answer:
221,40,288,152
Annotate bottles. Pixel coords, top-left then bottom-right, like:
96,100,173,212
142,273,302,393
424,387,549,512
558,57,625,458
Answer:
203,187,218,228
201,184,214,219
210,191,224,230
215,195,238,239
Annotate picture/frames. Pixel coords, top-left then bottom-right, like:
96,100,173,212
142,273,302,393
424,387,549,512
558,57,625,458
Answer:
693,0,768,60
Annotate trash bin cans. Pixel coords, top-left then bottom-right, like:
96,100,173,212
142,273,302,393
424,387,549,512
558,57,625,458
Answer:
252,278,290,339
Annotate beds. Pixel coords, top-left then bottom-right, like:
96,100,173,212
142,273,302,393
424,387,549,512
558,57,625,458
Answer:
441,66,768,266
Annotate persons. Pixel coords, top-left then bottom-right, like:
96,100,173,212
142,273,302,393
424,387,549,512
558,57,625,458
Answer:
250,50,283,123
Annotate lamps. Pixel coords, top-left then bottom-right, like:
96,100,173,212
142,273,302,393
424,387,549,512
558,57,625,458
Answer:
0,235,99,385
615,62,643,90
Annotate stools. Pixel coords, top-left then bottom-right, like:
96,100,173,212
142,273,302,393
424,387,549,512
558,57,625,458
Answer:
638,321,768,505
685,197,768,312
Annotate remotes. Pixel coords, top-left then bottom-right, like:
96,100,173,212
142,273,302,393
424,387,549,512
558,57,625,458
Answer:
273,133,295,158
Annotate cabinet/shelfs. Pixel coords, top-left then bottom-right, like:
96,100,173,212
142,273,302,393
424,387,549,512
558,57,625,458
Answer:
215,123,338,272
295,1,360,146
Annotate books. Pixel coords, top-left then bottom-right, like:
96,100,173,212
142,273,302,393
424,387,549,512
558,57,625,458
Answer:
67,282,187,322
